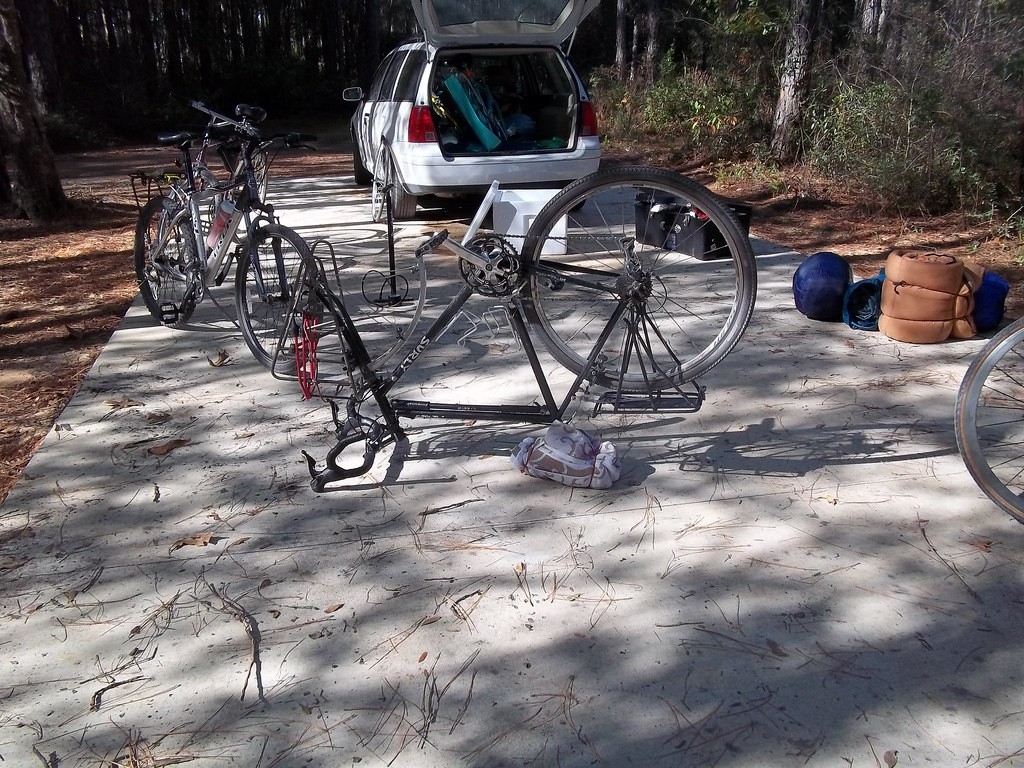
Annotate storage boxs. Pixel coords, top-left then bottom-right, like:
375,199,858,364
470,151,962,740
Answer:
462,179,569,257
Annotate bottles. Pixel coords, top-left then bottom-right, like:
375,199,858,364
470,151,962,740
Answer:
207,200,236,249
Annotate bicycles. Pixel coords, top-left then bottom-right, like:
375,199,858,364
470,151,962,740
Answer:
954,316,1024,528
124,100,323,373
270,165,756,493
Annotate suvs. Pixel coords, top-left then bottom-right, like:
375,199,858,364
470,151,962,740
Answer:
342,0,600,220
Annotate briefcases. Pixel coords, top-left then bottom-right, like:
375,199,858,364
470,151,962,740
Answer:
633,190,751,260
